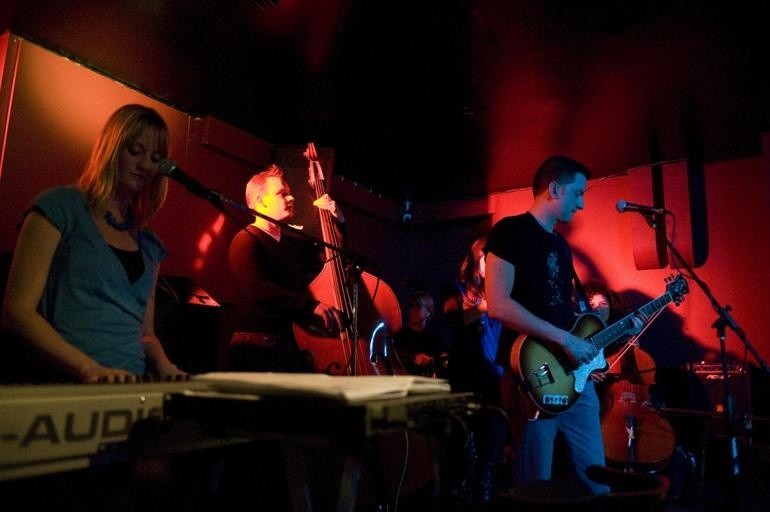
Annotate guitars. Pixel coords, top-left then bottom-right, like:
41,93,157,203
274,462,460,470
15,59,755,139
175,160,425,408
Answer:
509,274,688,413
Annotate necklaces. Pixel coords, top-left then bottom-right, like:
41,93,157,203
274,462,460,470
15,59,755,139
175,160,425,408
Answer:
101,202,136,232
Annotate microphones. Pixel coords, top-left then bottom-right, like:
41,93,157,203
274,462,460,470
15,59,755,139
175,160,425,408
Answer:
156,158,224,211
615,198,664,217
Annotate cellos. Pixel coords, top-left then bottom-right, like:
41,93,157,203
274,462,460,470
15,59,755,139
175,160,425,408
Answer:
601,286,677,473
293,143,440,512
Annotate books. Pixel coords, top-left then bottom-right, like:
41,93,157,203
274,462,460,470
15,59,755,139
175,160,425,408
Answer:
399,373,452,396
190,369,411,404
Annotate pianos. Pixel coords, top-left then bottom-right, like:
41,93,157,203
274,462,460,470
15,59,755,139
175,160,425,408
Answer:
0,382,472,481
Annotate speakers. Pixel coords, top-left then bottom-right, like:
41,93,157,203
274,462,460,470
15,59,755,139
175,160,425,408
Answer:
689,364,753,415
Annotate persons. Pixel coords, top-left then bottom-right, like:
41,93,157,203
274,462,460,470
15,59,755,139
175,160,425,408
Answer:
221,162,345,371
479,152,615,492
439,232,512,435
582,276,640,385
389,288,452,377
0,103,190,384
